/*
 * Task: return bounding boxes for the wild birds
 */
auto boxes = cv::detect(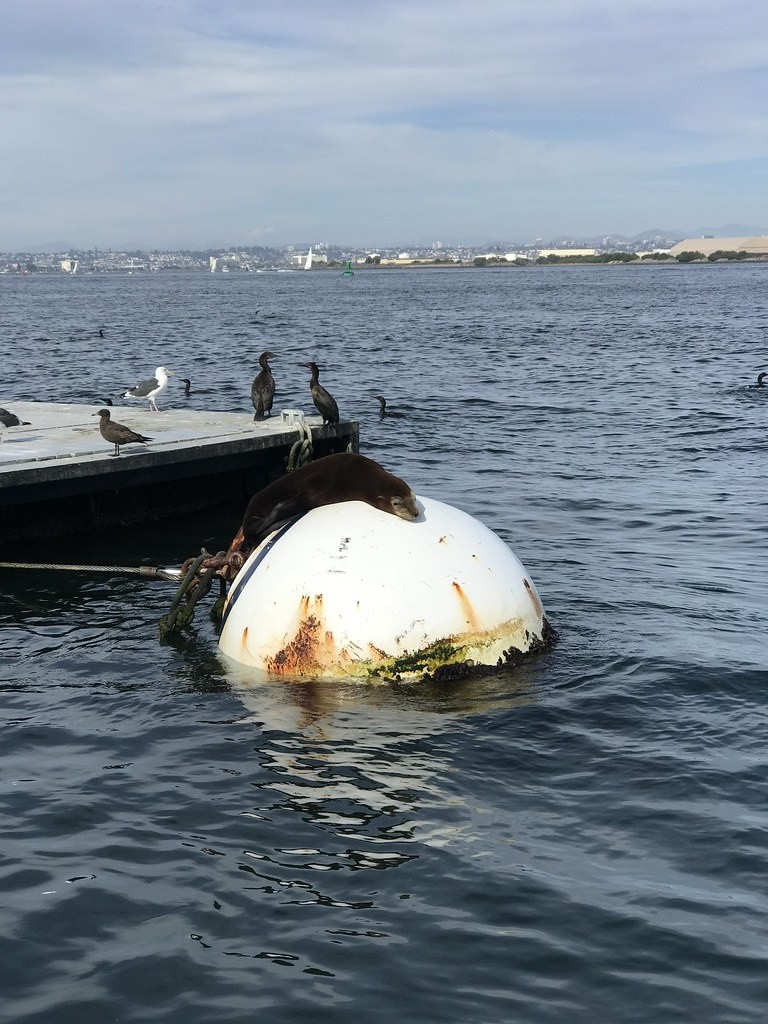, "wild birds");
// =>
[297,362,339,427]
[373,396,405,418]
[0,408,31,441]
[180,379,192,397]
[119,366,175,414]
[93,330,103,337]
[255,310,261,314]
[746,372,768,388]
[92,398,154,457]
[251,352,281,422]
